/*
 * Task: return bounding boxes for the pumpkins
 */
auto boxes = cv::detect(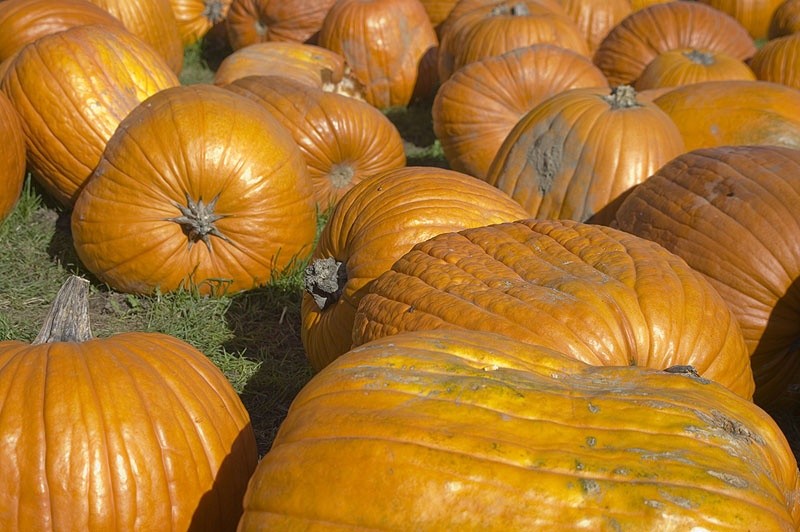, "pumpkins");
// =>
[0,1,798,532]
